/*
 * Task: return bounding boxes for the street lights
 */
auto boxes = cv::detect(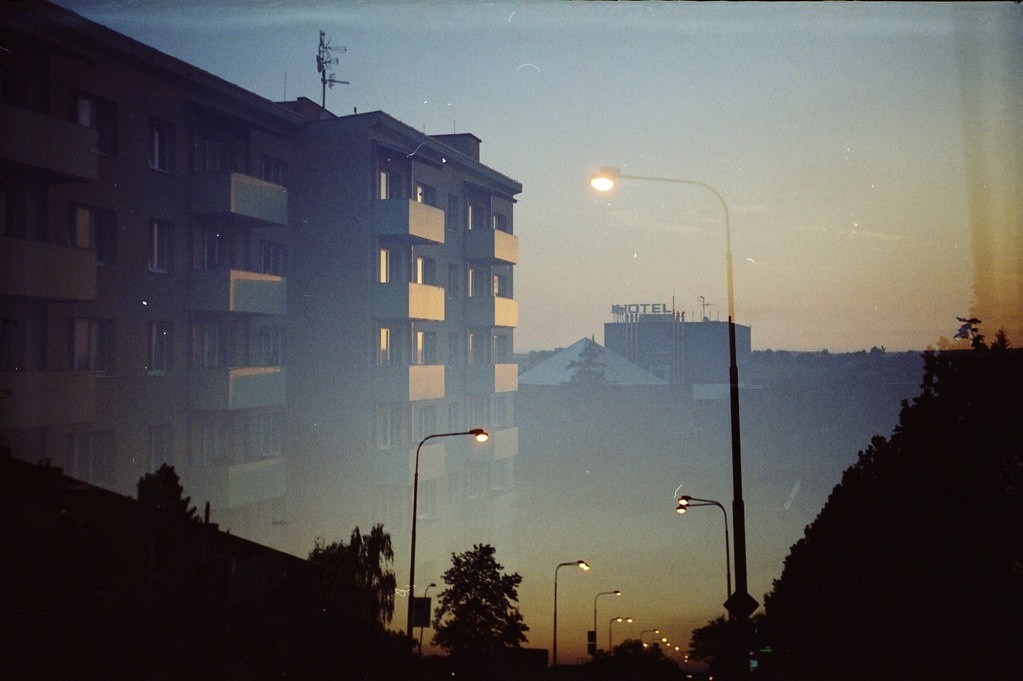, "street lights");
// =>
[551,560,590,681]
[675,494,734,626]
[589,172,750,681]
[402,428,490,681]
[592,589,689,674]
